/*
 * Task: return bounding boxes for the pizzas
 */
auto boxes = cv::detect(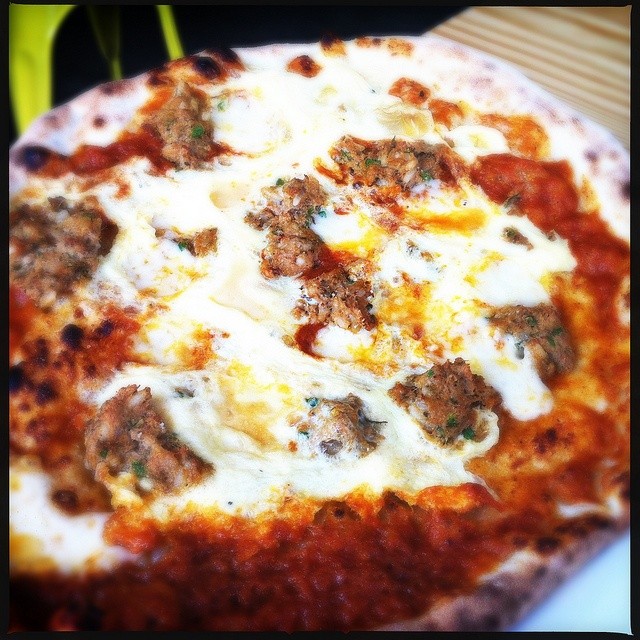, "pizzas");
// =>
[1,35,637,631]
